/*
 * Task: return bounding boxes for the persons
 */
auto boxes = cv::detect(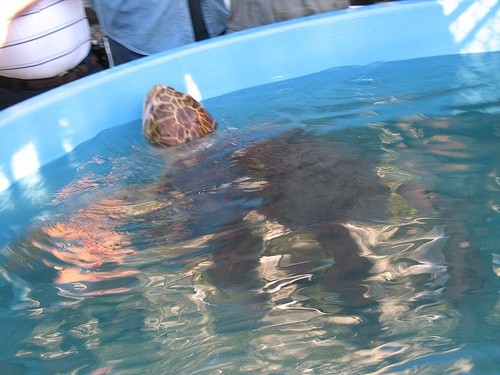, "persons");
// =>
[86,0,235,72]
[0,0,104,124]
